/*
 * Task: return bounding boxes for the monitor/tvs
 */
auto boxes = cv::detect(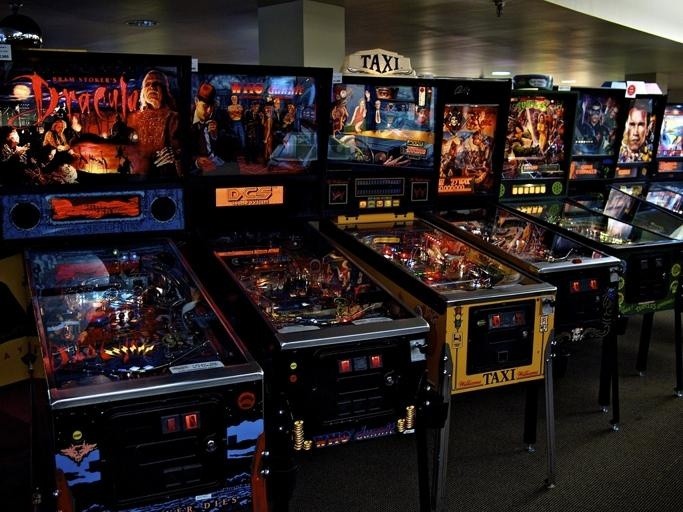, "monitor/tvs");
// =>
[1,55,682,185]
